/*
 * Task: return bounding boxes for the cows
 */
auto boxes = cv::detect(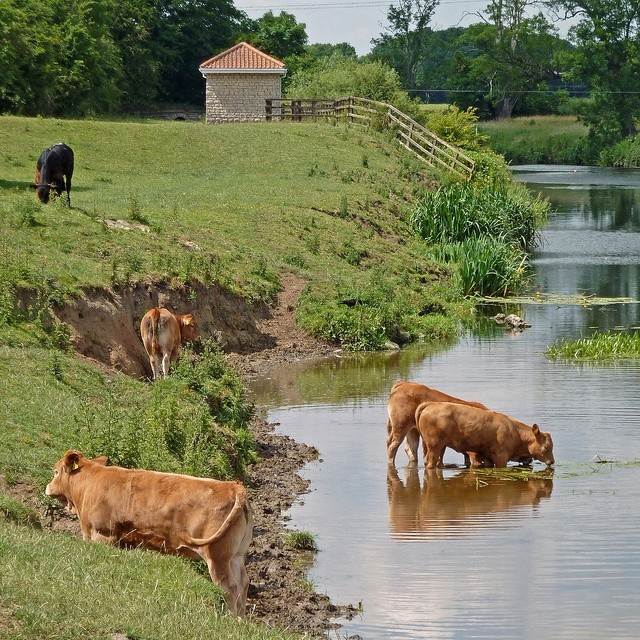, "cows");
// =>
[45,449,253,619]
[140,307,201,380]
[417,467,554,531]
[414,401,555,468]
[386,462,533,535]
[28,142,74,208]
[386,380,533,468]
